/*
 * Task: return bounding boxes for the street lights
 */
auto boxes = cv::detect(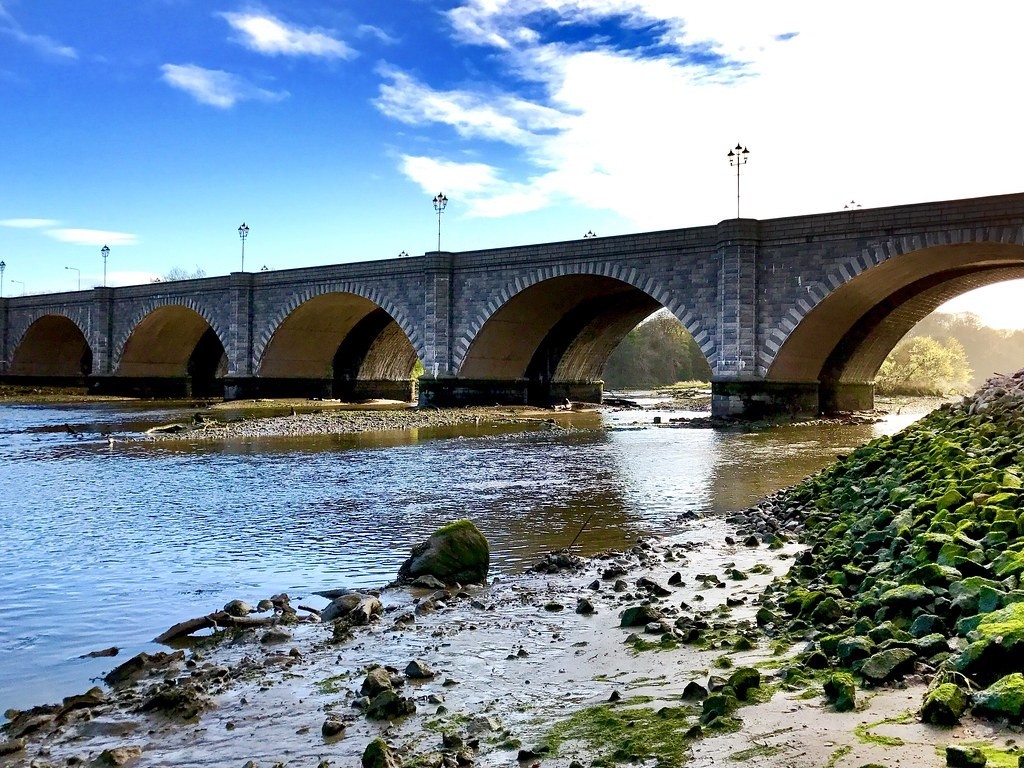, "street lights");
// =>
[728,143,751,218]
[433,191,448,250]
[0,260,6,297]
[65,266,80,292]
[11,280,24,296]
[101,245,110,283]
[238,221,250,271]
